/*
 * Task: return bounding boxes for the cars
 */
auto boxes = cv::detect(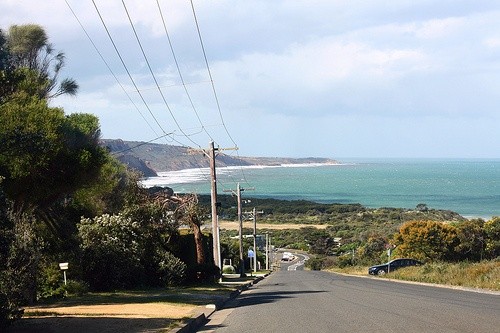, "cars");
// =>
[369,258,425,277]
